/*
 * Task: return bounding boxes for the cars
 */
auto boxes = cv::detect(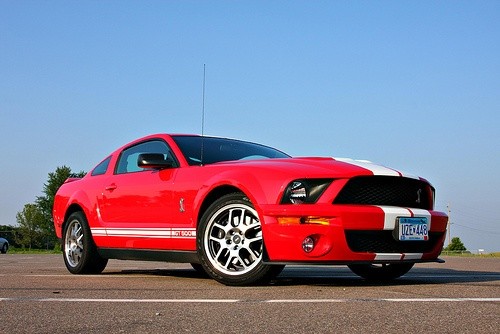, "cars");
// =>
[0,234,9,254]
[52,134,449,285]
